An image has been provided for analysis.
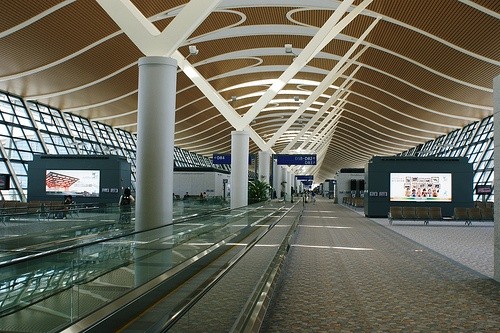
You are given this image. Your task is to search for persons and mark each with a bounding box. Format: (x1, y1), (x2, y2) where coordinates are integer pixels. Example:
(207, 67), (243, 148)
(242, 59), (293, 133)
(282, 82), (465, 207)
(173, 193), (177, 199)
(118, 188), (135, 224)
(54, 195), (74, 219)
(291, 187), (295, 203)
(304, 190), (316, 206)
(183, 192), (190, 200)
(405, 187), (438, 197)
(200, 192), (209, 203)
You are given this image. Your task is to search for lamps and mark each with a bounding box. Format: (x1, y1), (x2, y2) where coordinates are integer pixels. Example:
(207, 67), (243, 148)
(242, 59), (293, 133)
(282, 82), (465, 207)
(293, 96), (299, 101)
(253, 121), (256, 123)
(232, 95), (236, 101)
(186, 45), (199, 60)
(284, 43), (299, 60)
(299, 120), (302, 123)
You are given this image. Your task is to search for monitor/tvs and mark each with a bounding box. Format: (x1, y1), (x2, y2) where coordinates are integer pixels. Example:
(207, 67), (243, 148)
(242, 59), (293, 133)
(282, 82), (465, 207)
(0, 174), (10, 190)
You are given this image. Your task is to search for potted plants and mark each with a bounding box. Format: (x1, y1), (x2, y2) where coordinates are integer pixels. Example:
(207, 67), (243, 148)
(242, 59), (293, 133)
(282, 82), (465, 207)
(249, 180), (269, 204)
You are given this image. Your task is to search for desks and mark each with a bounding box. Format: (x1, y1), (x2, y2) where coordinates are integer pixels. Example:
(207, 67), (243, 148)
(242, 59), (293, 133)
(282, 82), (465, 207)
(0, 206), (42, 226)
(44, 204), (82, 220)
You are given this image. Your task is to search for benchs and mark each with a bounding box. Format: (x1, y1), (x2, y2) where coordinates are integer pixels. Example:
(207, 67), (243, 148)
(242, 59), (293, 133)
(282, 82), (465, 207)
(174, 195), (181, 200)
(1, 206), (99, 225)
(454, 202), (494, 226)
(343, 197), (365, 210)
(387, 207), (443, 226)
(185, 195), (200, 201)
